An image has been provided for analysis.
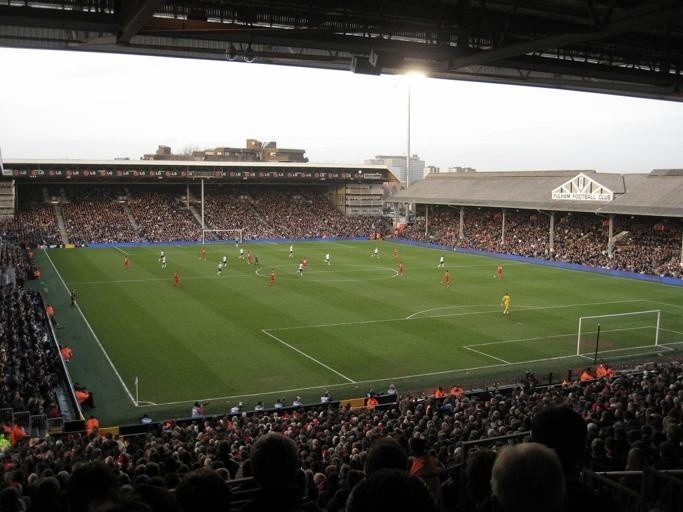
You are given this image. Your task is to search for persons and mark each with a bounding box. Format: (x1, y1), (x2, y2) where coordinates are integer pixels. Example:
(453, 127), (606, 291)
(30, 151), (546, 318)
(123, 256), (128, 268)
(296, 259), (306, 276)
(500, 292), (511, 316)
(1, 377), (682, 512)
(271, 271), (275, 283)
(393, 249), (397, 256)
(289, 245), (293, 259)
(216, 255), (227, 276)
(17, 172), (394, 245)
(398, 207), (683, 286)
(158, 250), (179, 283)
(235, 237), (260, 266)
(325, 252), (331, 266)
(1, 216), (42, 292)
(373, 248), (378, 257)
(200, 249), (206, 259)
(0, 285), (61, 377)
